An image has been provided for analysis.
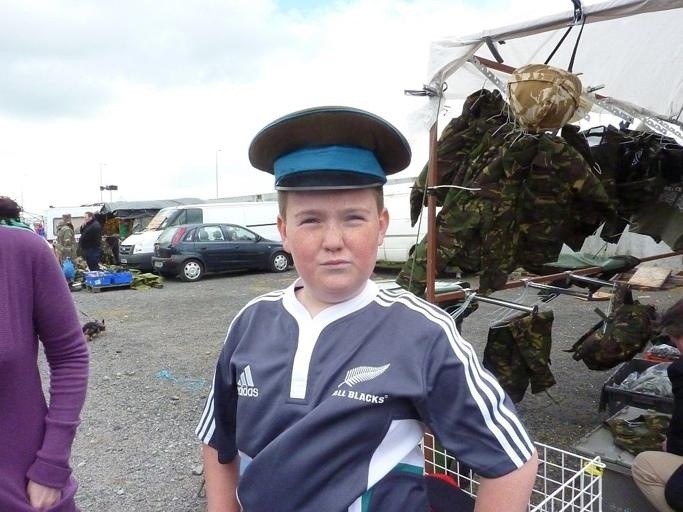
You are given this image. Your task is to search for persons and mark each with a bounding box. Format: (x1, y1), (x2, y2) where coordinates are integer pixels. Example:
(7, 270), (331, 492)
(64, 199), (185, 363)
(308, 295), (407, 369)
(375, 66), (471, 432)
(195, 104), (538, 512)
(80, 212), (102, 271)
(631, 300), (683, 512)
(36, 224), (45, 237)
(57, 214), (77, 284)
(0, 196), (92, 511)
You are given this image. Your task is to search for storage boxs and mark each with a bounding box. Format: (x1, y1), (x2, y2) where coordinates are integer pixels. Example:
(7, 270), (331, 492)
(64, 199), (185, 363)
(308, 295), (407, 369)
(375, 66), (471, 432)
(600, 358), (673, 418)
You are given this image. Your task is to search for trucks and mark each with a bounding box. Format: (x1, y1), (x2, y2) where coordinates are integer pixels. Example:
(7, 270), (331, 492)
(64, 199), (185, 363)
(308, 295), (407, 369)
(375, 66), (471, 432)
(374, 182), (444, 269)
(45, 205), (104, 248)
(116, 201), (280, 272)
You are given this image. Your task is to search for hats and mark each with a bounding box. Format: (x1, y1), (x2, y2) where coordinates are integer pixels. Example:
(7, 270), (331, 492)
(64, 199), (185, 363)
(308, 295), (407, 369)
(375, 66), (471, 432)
(249, 106), (410, 191)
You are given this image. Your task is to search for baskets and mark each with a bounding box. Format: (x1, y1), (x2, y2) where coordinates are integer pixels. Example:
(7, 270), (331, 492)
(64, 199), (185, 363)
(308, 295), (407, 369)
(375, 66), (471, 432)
(424, 421), (603, 512)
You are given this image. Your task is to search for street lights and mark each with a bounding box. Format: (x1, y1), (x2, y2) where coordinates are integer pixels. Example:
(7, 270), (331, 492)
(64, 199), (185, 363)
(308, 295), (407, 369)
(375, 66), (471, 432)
(99, 184), (118, 203)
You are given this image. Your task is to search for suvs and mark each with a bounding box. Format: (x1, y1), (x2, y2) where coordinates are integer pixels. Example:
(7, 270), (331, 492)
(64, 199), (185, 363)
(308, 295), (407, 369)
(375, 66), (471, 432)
(151, 223), (293, 282)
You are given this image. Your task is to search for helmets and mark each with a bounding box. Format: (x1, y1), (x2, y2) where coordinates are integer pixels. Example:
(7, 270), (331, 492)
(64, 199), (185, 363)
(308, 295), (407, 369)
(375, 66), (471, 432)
(507, 65), (582, 131)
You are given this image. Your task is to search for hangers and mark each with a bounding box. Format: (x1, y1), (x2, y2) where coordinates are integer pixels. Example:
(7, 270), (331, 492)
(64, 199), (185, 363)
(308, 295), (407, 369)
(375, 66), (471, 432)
(592, 209), (639, 239)
(444, 271), (556, 334)
(445, 75), (683, 175)
(601, 279), (618, 335)
